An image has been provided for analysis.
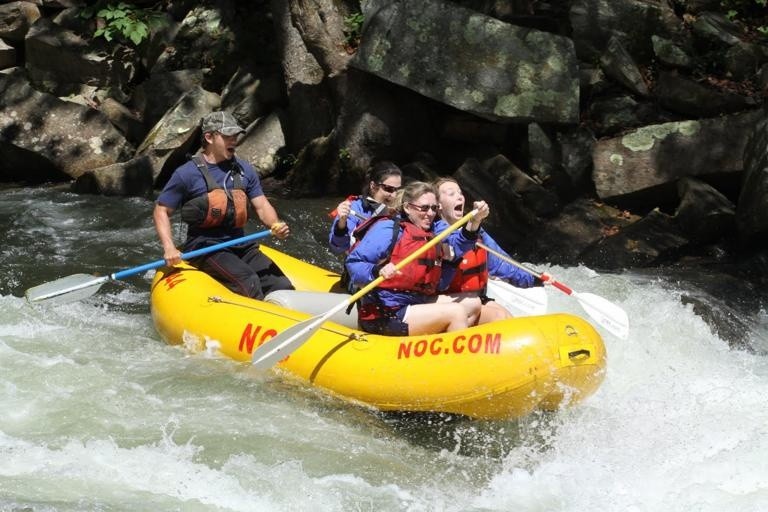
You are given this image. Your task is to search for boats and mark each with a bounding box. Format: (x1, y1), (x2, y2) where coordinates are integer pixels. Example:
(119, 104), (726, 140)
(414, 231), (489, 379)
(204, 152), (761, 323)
(144, 243), (609, 426)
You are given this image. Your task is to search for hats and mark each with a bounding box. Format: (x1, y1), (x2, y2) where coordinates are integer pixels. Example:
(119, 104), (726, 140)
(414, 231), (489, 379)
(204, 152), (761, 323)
(202, 111), (246, 136)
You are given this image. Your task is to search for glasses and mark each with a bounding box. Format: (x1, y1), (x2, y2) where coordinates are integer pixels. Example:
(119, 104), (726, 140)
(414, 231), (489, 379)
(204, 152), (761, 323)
(377, 182), (404, 193)
(408, 203), (438, 212)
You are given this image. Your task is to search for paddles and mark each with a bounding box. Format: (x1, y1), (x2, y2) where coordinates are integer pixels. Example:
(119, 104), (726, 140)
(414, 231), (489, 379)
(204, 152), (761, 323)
(474, 242), (631, 341)
(25, 221), (283, 313)
(251, 207), (479, 374)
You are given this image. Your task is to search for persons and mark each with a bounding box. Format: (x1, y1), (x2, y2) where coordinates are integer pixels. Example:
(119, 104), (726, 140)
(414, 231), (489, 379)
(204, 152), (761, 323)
(327, 159), (404, 293)
(429, 174), (554, 323)
(341, 181), (490, 335)
(154, 109), (294, 306)
